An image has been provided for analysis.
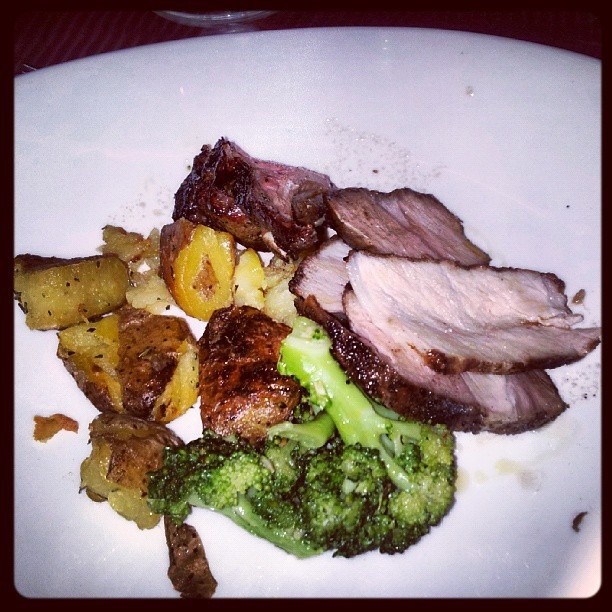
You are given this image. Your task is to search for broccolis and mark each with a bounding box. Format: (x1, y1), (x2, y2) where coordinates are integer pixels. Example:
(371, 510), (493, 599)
(146, 317), (457, 557)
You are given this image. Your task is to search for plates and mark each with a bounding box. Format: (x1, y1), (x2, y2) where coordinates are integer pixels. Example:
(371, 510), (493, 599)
(13, 25), (604, 600)
